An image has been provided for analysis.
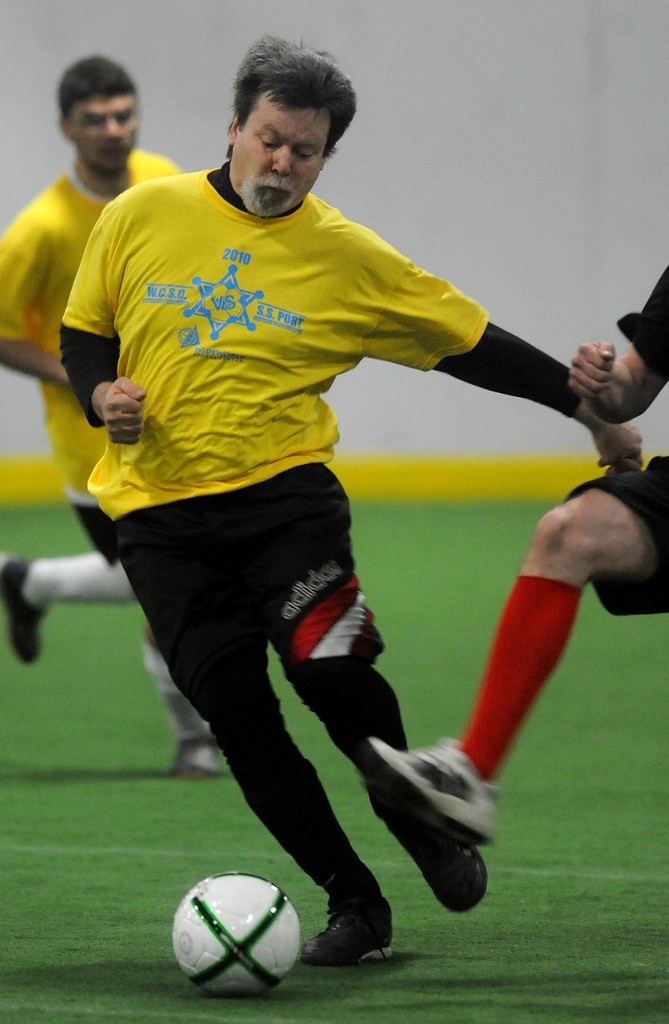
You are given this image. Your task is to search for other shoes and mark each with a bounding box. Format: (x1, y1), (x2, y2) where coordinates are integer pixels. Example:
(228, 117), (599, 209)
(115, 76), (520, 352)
(401, 821), (488, 911)
(298, 906), (395, 966)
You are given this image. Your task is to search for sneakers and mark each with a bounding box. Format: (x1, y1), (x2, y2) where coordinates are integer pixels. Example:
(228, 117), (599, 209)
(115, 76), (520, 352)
(361, 736), (501, 845)
(171, 739), (223, 777)
(0, 553), (40, 662)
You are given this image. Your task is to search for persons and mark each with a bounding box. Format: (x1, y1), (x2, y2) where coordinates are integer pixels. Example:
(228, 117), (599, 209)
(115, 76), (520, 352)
(361, 265), (668, 850)
(1, 52), (226, 779)
(57, 30), (647, 970)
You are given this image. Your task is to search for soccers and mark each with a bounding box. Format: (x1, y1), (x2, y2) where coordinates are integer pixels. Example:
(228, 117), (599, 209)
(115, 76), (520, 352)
(172, 870), (301, 997)
